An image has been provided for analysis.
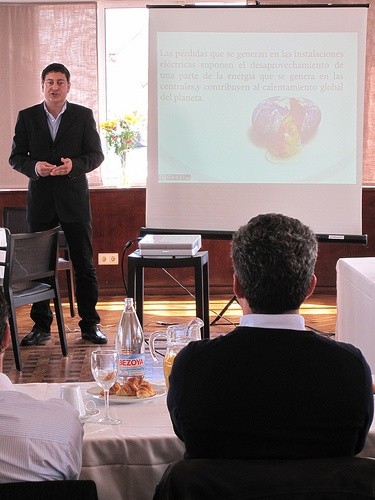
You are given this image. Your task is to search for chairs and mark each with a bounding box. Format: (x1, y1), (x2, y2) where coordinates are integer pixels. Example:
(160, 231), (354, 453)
(0, 207), (75, 371)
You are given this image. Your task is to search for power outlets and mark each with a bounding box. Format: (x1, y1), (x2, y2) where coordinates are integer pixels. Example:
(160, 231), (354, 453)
(98, 252), (119, 265)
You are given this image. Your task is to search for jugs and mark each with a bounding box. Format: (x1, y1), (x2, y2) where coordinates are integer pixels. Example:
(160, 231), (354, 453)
(149, 318), (204, 387)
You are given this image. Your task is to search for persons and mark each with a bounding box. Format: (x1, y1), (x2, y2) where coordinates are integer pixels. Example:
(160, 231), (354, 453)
(8, 63), (108, 346)
(166, 213), (374, 459)
(0, 285), (84, 483)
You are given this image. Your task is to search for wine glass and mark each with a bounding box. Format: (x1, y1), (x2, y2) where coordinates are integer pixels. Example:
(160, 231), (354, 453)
(91, 350), (123, 425)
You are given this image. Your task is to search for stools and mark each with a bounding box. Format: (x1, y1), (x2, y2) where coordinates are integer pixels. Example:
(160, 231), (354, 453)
(127, 249), (211, 342)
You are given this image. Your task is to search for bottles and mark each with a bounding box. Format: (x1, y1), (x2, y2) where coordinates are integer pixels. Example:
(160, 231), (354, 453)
(114, 297), (145, 385)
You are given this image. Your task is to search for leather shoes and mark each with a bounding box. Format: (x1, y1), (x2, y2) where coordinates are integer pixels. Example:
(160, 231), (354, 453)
(21, 328), (51, 346)
(81, 328), (108, 344)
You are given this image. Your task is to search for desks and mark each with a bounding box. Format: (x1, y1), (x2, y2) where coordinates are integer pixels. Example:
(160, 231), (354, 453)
(12, 380), (187, 500)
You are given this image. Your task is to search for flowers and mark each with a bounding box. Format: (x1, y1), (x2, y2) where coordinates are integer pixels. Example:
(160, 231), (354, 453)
(98, 114), (140, 167)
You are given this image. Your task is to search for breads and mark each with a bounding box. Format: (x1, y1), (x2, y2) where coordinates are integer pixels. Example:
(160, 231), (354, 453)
(100, 372), (155, 398)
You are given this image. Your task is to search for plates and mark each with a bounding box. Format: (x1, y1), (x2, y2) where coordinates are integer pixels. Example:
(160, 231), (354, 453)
(88, 384), (166, 404)
(80, 409), (100, 420)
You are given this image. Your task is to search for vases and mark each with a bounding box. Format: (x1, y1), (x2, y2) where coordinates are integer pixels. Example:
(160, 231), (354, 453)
(118, 148), (128, 187)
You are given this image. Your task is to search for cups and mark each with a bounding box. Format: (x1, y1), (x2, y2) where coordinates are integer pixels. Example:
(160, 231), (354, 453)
(61, 385), (87, 416)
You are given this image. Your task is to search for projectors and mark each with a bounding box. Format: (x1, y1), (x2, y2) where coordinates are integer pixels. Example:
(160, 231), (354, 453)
(139, 235), (201, 256)
